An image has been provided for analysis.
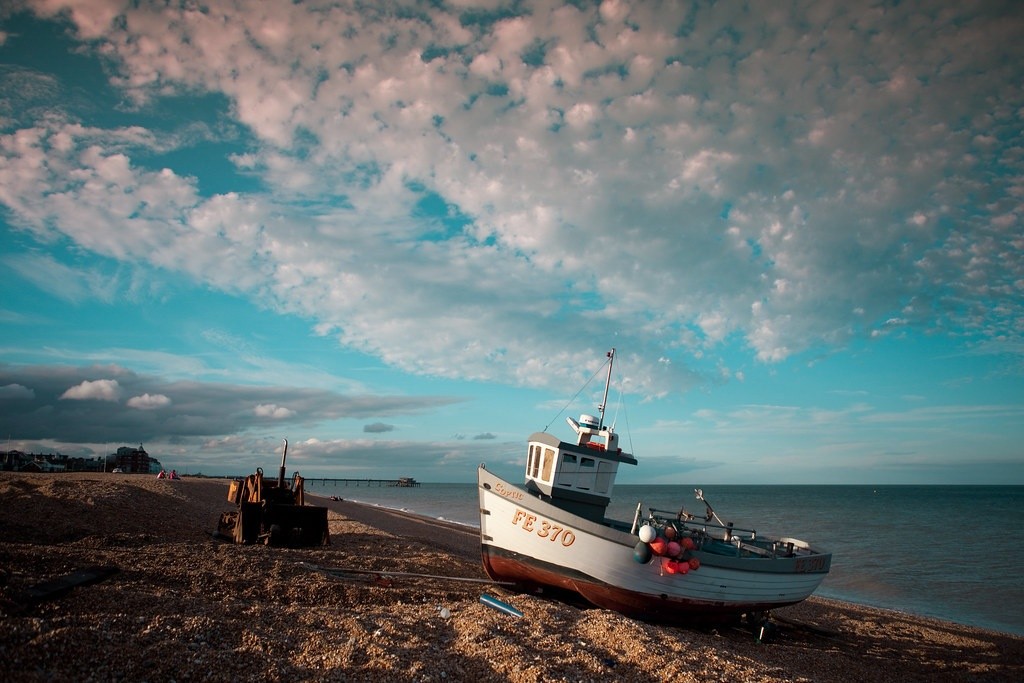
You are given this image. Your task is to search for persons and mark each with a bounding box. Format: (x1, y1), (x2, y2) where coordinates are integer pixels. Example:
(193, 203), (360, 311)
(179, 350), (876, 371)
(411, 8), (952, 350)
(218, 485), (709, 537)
(156, 469), (167, 479)
(169, 469), (179, 479)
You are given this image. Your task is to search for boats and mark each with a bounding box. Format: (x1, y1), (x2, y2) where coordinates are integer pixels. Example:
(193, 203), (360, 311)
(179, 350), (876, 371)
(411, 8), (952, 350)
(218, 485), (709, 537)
(478, 349), (833, 628)
(400, 477), (416, 483)
(113, 468), (123, 474)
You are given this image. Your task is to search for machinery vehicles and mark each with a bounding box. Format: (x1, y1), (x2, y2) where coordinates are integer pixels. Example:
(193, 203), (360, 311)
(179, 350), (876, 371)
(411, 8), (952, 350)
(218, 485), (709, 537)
(212, 438), (329, 549)
